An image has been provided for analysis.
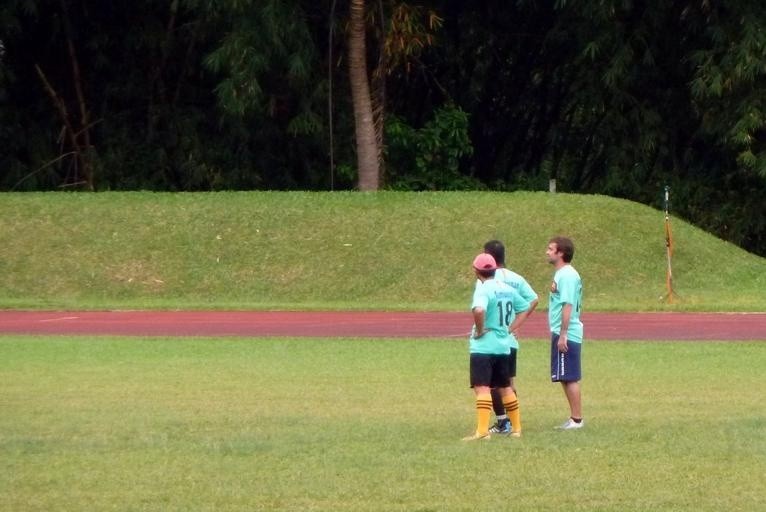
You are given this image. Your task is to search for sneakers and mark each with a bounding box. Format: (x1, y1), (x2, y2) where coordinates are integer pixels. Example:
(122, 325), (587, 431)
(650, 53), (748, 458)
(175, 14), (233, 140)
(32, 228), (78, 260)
(506, 430), (522, 439)
(487, 416), (513, 435)
(552, 416), (584, 432)
(458, 430), (492, 442)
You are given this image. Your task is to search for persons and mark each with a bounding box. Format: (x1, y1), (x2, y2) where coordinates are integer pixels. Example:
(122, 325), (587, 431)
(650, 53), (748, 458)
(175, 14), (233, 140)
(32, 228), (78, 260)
(546, 237), (586, 430)
(460, 253), (530, 443)
(475, 239), (539, 436)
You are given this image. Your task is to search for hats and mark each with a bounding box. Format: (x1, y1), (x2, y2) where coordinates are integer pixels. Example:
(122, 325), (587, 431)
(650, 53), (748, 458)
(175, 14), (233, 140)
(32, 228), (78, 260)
(471, 252), (499, 272)
